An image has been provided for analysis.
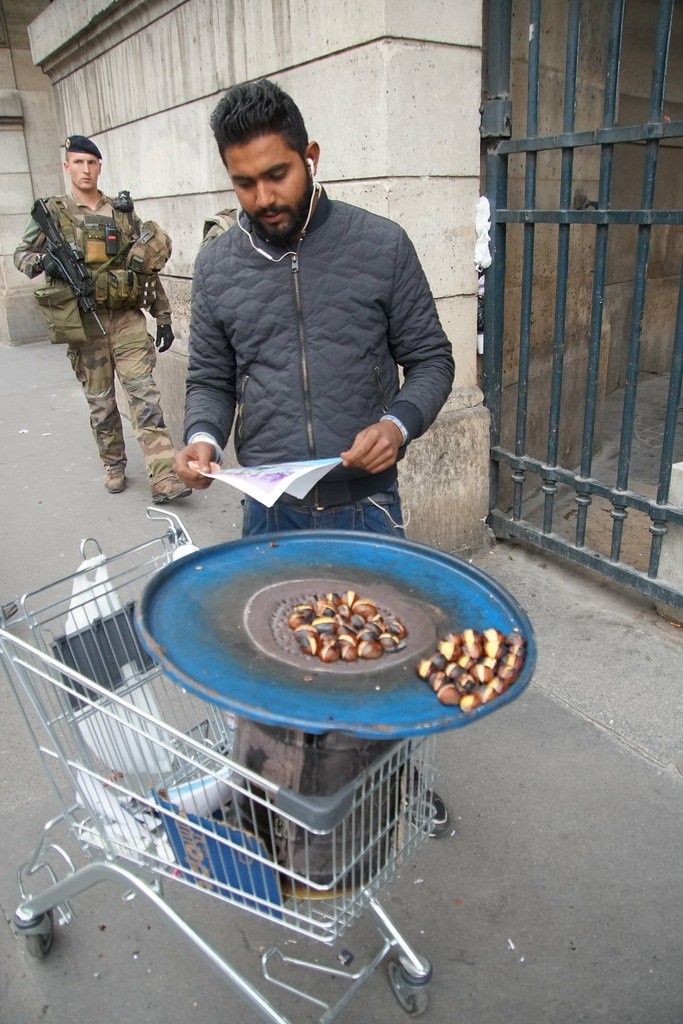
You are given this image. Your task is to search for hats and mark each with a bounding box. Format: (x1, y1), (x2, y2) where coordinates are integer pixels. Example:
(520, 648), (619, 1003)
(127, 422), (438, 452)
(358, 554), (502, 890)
(65, 135), (102, 160)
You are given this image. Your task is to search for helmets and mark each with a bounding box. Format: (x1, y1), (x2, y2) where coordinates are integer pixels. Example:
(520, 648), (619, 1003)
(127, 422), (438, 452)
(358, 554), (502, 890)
(126, 221), (173, 275)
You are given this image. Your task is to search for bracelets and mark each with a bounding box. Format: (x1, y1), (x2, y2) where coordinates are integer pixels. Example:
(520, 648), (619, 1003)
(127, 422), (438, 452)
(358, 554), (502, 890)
(189, 436), (224, 465)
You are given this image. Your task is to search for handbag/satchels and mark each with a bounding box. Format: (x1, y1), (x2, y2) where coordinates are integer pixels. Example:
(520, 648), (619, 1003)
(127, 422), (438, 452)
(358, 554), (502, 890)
(34, 284), (87, 344)
(65, 554), (176, 775)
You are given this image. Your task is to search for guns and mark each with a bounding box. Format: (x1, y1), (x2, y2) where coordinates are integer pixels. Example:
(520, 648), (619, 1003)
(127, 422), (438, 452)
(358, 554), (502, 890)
(30, 197), (109, 336)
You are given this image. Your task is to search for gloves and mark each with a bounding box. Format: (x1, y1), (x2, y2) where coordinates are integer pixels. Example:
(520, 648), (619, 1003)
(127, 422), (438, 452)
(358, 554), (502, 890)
(41, 253), (68, 282)
(156, 324), (174, 353)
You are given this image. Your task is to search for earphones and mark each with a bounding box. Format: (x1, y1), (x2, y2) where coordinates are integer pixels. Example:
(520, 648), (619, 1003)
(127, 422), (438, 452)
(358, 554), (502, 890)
(307, 158), (314, 174)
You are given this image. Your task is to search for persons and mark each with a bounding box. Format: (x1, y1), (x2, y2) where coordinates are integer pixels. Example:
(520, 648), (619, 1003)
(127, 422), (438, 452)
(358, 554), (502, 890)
(173, 80), (455, 838)
(13, 135), (192, 504)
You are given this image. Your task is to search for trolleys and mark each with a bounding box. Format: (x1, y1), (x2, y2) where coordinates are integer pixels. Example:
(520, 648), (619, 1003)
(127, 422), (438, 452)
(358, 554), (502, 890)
(0, 507), (438, 1024)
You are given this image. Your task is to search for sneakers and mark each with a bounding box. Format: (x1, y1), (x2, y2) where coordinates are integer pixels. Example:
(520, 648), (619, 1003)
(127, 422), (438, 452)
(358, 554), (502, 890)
(103, 467), (126, 493)
(150, 477), (193, 503)
(398, 764), (451, 837)
